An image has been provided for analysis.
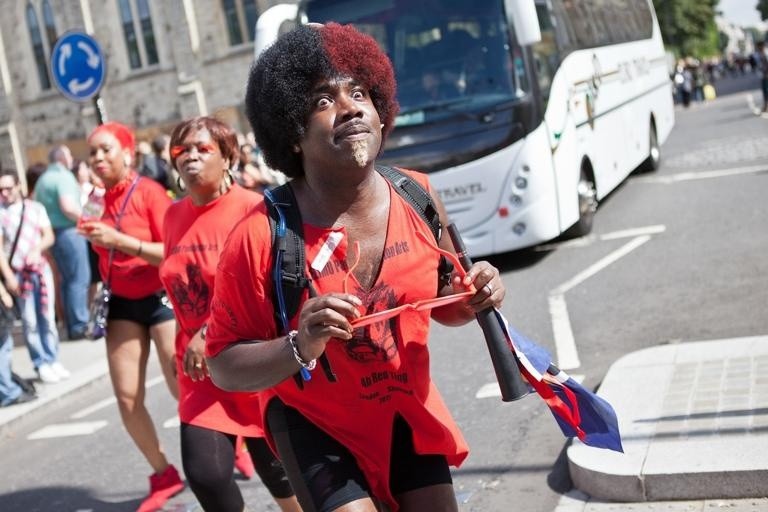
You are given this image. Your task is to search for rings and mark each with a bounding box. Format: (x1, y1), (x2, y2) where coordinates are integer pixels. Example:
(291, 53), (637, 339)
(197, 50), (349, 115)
(483, 282), (494, 294)
(195, 362), (202, 369)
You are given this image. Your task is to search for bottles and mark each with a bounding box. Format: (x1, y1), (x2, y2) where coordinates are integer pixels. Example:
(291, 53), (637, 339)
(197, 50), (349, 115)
(75, 185), (107, 241)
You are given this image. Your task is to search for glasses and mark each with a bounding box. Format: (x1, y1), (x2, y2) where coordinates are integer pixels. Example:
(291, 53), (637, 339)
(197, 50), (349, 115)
(342, 231), (478, 330)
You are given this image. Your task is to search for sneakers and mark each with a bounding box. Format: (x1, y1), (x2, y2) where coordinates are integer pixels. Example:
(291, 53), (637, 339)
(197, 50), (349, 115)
(137, 466), (183, 512)
(236, 446), (253, 479)
(10, 363), (68, 403)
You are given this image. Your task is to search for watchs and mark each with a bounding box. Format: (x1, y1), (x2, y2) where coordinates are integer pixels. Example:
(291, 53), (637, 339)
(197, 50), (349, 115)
(198, 323), (211, 337)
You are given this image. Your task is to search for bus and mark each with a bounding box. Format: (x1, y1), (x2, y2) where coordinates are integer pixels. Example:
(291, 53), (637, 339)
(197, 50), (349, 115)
(255, 0), (676, 259)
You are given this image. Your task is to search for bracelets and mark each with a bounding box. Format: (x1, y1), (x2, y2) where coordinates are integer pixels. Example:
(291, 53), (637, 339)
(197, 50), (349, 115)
(135, 238), (144, 257)
(285, 327), (318, 372)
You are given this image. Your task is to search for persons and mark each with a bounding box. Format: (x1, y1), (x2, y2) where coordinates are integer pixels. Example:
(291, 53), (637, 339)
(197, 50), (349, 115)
(669, 39), (768, 117)
(1, 169), (73, 405)
(77, 120), (255, 511)
(154, 115), (302, 511)
(27, 134), (286, 340)
(198, 19), (510, 512)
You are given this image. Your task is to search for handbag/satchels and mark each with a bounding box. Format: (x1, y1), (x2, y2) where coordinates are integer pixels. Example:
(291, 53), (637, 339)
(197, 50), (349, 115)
(86, 288), (110, 339)
